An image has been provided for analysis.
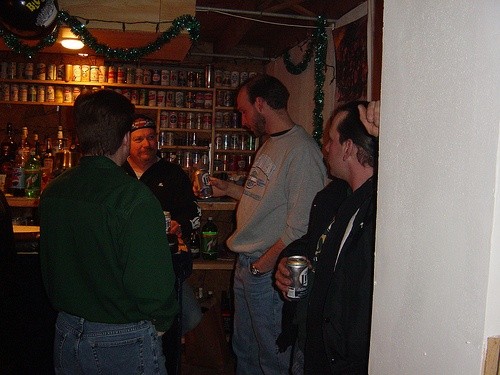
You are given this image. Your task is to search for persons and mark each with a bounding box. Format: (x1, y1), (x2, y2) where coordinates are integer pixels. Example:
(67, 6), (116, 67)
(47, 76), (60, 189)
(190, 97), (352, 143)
(39, 90), (179, 375)
(120, 114), (198, 375)
(274, 99), (381, 375)
(192, 74), (325, 375)
(0, 189), (18, 375)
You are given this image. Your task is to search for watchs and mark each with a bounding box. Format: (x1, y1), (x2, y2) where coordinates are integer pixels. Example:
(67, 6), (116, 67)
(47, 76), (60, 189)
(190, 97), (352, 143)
(250, 263), (267, 276)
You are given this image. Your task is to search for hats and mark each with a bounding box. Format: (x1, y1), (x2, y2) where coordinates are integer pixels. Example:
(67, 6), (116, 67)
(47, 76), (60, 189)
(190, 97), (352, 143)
(129, 113), (158, 133)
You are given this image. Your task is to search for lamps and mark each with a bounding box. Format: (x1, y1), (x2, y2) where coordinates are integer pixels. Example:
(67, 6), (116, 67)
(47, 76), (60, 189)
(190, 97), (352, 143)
(60, 29), (84, 50)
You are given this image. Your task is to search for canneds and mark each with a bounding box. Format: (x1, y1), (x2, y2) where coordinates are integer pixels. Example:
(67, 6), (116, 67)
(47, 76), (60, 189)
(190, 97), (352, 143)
(160, 131), (261, 171)
(164, 212), (171, 234)
(0, 60), (257, 109)
(160, 111), (242, 130)
(195, 168), (213, 198)
(285, 255), (309, 299)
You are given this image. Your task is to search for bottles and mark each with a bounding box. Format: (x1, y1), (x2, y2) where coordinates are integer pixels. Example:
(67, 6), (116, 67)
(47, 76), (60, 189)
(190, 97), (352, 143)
(1, 122), (80, 199)
(189, 229), (200, 257)
(201, 217), (219, 259)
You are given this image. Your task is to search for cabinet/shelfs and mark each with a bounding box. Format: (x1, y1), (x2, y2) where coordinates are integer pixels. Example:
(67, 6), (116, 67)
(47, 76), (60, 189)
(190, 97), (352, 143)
(0, 78), (258, 178)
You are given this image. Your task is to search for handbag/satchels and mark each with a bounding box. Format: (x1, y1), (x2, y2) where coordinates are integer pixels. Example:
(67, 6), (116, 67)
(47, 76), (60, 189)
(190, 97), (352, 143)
(183, 299), (232, 369)
